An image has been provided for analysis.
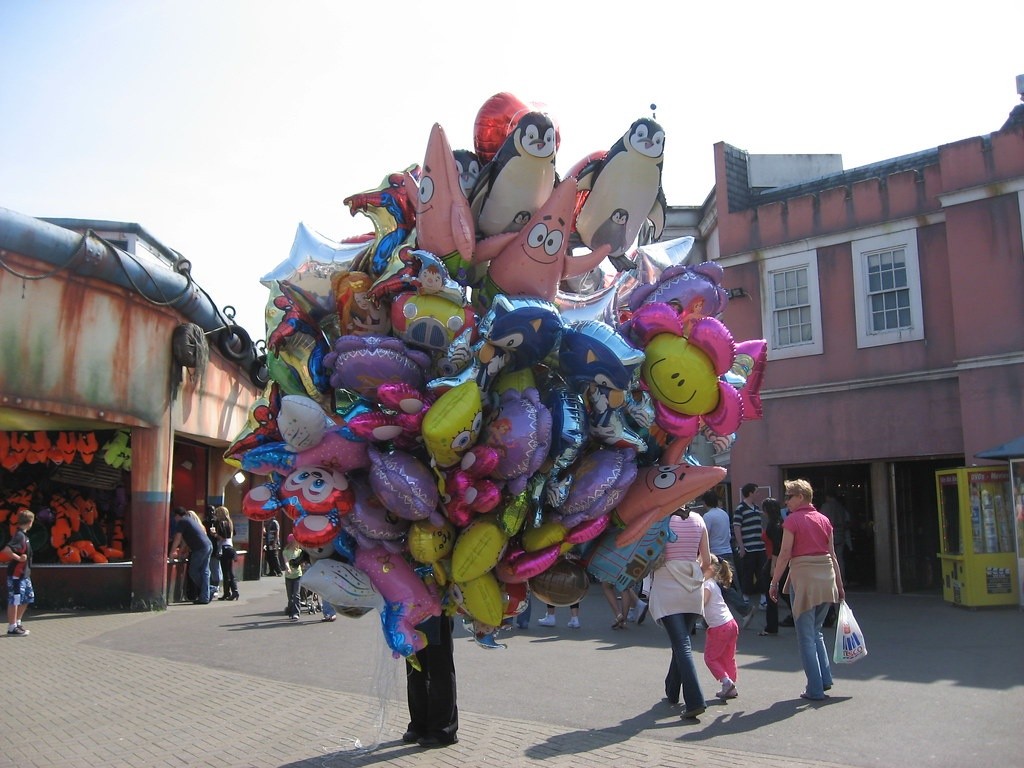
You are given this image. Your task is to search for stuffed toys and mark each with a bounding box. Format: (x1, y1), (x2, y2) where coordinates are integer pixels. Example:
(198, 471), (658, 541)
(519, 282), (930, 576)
(0, 429), (131, 563)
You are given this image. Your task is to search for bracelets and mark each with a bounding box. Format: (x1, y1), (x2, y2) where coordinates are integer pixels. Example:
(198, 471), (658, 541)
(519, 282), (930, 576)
(770, 583), (778, 588)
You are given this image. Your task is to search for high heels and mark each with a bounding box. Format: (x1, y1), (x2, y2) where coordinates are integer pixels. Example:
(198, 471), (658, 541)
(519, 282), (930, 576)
(226, 591), (239, 600)
(218, 592), (231, 600)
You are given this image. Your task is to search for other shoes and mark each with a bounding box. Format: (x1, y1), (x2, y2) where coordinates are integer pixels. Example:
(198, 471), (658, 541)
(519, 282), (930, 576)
(611, 614), (626, 628)
(738, 594), (795, 636)
(7, 625), (30, 637)
(194, 600), (208, 604)
(681, 707), (706, 717)
(715, 682), (738, 699)
(322, 613), (336, 621)
(696, 622), (707, 629)
(418, 735), (447, 747)
(402, 730), (424, 742)
(800, 690), (809, 698)
(636, 602), (648, 624)
(268, 572), (277, 576)
(823, 683), (831, 691)
(627, 615), (635, 622)
(277, 572), (283, 577)
(289, 613), (299, 620)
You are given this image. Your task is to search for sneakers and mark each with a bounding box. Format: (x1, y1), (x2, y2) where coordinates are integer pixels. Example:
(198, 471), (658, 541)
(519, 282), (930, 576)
(538, 616), (556, 626)
(567, 620), (580, 627)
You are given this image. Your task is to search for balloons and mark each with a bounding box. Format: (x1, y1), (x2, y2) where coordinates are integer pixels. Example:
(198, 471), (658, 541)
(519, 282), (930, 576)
(218, 92), (771, 671)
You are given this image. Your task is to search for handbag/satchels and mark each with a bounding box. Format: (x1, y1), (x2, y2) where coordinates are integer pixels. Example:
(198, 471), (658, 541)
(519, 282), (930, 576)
(833, 600), (868, 663)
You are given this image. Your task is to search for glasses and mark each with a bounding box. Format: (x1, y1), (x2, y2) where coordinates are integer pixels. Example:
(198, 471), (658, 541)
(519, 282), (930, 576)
(785, 494), (798, 501)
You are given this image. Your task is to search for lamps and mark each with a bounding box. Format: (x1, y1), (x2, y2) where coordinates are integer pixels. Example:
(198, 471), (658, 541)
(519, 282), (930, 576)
(731, 288), (745, 297)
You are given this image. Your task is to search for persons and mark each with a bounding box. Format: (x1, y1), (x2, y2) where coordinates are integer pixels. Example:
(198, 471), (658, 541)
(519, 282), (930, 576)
(282, 534), (337, 622)
(644, 481), (797, 720)
(263, 516), (283, 577)
(403, 608), (460, 747)
(3, 509), (34, 637)
(503, 579), (649, 629)
(769, 478), (844, 701)
(169, 505), (239, 604)
